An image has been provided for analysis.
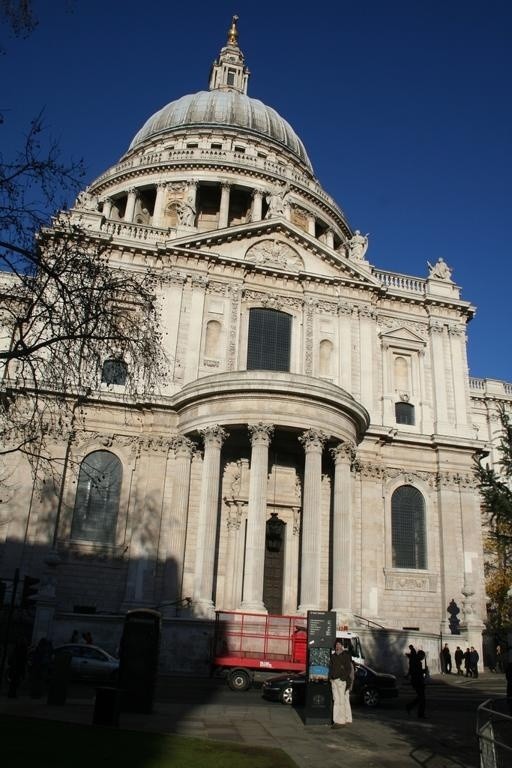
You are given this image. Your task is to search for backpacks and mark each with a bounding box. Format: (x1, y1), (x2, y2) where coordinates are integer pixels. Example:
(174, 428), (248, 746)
(348, 661), (354, 691)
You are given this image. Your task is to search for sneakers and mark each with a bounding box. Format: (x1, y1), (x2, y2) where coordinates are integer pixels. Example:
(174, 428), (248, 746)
(331, 722), (352, 729)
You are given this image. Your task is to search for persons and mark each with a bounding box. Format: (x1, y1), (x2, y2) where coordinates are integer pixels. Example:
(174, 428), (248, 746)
(349, 229), (367, 261)
(427, 256), (451, 280)
(405, 644), (416, 676)
(469, 646), (479, 679)
(454, 647), (464, 675)
(341, 648), (356, 723)
(328, 643), (351, 730)
(442, 643), (452, 674)
(410, 649), (429, 718)
(177, 196), (198, 227)
(462, 648), (472, 677)
(265, 180), (287, 218)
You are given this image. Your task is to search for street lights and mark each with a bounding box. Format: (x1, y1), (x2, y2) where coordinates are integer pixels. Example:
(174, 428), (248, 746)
(50, 389), (90, 549)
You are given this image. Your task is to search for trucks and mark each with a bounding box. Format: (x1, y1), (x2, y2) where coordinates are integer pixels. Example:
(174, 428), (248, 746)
(214, 610), (368, 692)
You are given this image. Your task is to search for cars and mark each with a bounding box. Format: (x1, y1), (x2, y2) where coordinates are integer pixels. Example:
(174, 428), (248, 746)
(261, 663), (400, 708)
(44, 643), (119, 673)
